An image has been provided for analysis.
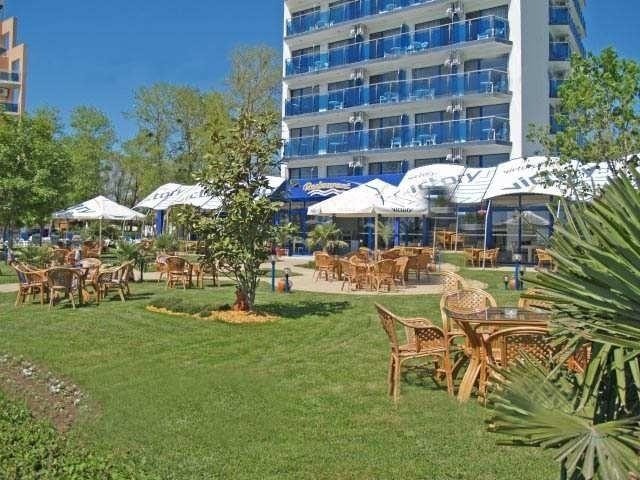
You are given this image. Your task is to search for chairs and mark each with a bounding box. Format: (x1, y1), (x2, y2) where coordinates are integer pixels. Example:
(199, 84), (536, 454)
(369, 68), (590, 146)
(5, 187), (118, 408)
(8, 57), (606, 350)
(463, 245), (500, 267)
(535, 248), (554, 270)
(440, 287), (592, 404)
(431, 228), (464, 252)
(315, 243), (435, 296)
(372, 302), (457, 404)
(11, 240), (219, 311)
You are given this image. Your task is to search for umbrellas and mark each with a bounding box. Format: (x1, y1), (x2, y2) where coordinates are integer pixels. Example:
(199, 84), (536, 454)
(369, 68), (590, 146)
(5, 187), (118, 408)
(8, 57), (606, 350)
(49, 194), (147, 256)
(304, 175), (436, 256)
